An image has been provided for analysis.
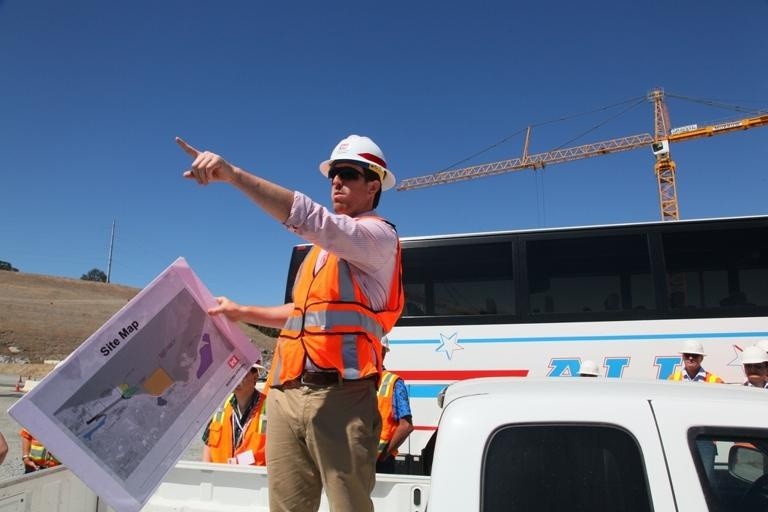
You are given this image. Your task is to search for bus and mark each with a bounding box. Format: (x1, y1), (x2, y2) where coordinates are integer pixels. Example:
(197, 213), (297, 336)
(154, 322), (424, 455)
(282, 213), (768, 467)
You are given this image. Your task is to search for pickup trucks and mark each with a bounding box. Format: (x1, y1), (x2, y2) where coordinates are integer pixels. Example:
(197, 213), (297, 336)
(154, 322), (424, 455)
(0, 371), (768, 511)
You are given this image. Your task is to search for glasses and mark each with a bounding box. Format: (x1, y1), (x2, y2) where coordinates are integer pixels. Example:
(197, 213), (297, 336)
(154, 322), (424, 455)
(328, 165), (365, 182)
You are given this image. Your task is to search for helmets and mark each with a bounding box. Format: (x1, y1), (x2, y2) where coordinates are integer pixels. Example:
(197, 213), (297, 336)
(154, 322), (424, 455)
(577, 360), (601, 376)
(678, 341), (712, 356)
(742, 348), (768, 364)
(320, 135), (396, 192)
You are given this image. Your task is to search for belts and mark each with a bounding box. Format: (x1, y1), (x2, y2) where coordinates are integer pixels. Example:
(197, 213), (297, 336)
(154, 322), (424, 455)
(281, 371), (341, 389)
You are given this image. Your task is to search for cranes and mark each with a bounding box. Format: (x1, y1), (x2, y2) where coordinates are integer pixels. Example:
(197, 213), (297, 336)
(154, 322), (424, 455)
(394, 84), (768, 222)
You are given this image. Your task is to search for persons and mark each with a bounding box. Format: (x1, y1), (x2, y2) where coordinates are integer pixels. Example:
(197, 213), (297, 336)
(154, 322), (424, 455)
(733, 347), (768, 449)
(19, 428), (59, 475)
(376, 333), (414, 474)
(201, 354), (268, 466)
(667, 340), (724, 483)
(172, 133), (405, 512)
(0, 432), (8, 465)
